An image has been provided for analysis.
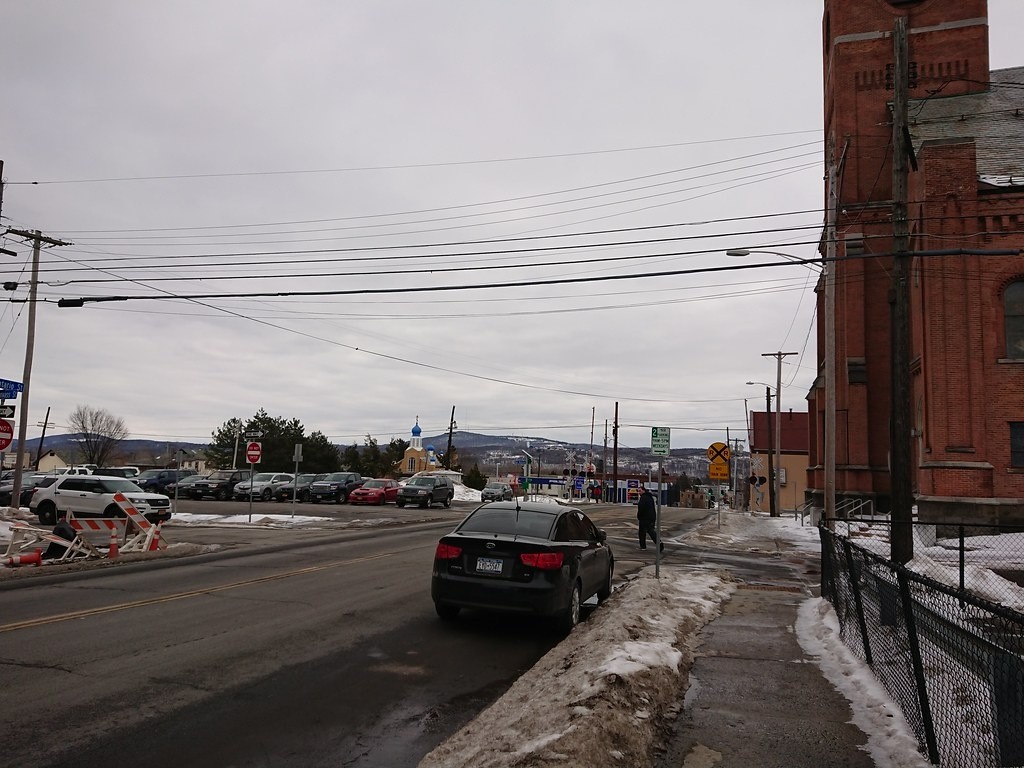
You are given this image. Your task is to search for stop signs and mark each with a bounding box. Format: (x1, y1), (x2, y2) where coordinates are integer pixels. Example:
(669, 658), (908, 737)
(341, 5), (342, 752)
(247, 443), (260, 462)
(0, 419), (13, 450)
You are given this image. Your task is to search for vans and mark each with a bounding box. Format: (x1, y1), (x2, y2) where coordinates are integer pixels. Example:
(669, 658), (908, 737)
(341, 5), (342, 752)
(128, 469), (199, 494)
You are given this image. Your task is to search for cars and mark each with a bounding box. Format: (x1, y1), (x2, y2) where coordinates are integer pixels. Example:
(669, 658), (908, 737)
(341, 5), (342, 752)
(430, 502), (614, 628)
(481, 482), (514, 502)
(163, 475), (207, 498)
(233, 472), (316, 502)
(349, 478), (402, 505)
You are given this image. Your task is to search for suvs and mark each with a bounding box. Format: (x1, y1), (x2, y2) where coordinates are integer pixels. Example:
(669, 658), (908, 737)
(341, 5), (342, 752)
(309, 472), (364, 504)
(189, 468), (259, 502)
(395, 473), (455, 508)
(0, 464), (173, 525)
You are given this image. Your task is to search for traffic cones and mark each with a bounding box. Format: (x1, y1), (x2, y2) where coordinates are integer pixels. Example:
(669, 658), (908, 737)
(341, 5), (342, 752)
(0, 547), (42, 567)
(149, 520), (163, 551)
(107, 526), (122, 558)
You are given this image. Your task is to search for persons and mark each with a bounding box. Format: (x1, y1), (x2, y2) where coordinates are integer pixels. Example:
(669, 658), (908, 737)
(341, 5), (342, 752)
(636, 487), (664, 552)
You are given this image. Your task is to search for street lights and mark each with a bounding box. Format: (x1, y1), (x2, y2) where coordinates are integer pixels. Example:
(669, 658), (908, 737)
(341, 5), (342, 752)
(745, 381), (782, 517)
(726, 249), (838, 532)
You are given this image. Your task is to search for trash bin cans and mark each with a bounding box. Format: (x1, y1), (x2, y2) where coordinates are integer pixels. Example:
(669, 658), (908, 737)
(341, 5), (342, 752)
(633, 496), (639, 505)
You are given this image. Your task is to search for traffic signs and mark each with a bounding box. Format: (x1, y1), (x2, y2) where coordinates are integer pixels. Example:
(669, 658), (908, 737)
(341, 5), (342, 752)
(0, 404), (15, 418)
(651, 427), (671, 456)
(244, 429), (265, 439)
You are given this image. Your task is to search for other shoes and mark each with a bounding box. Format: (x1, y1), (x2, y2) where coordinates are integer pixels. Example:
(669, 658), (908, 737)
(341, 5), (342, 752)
(637, 548), (647, 550)
(659, 542), (665, 552)
